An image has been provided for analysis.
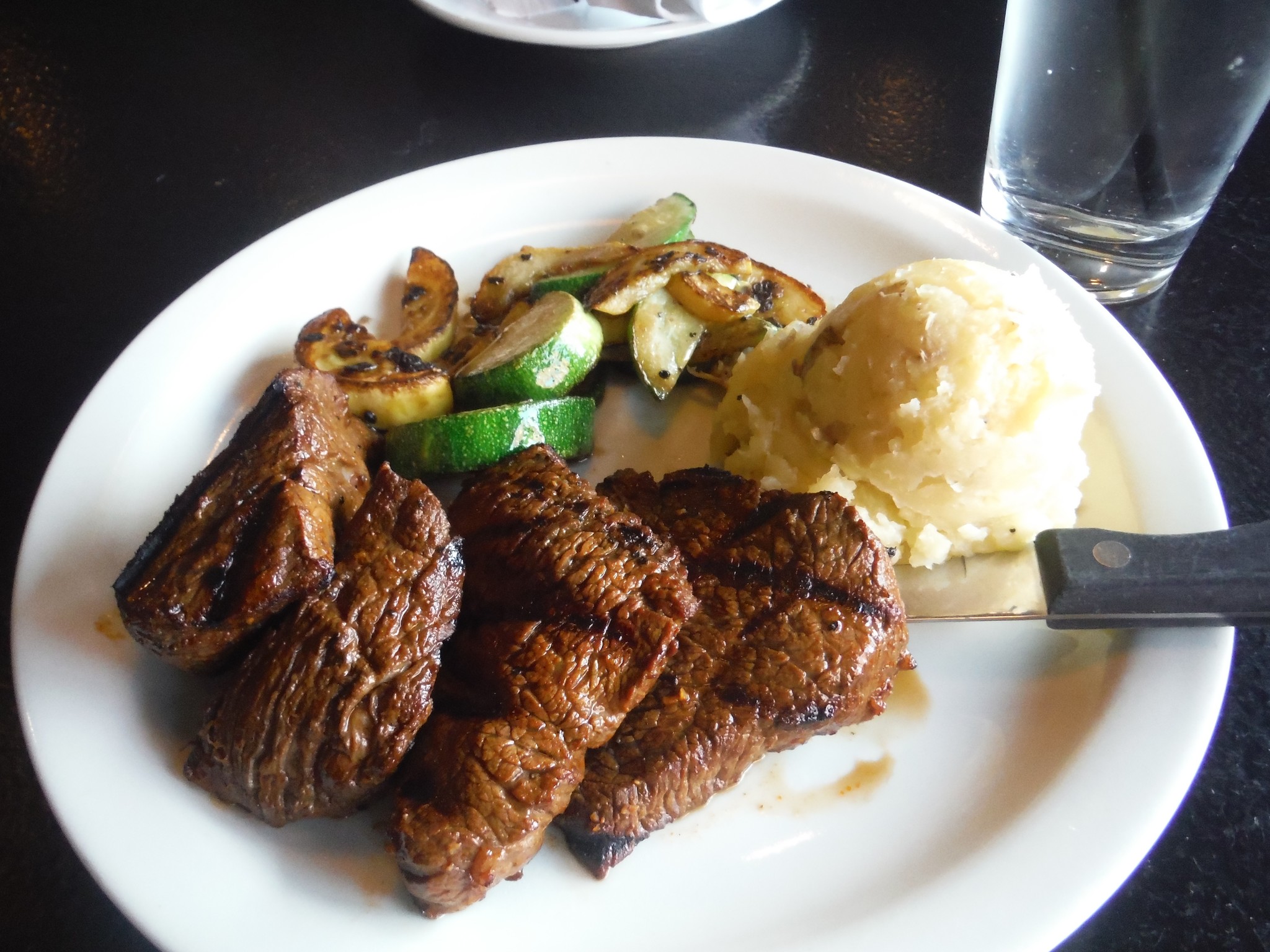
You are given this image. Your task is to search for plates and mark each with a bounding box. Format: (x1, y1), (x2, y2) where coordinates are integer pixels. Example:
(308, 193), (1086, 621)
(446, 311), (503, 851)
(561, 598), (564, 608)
(414, 1), (783, 49)
(10, 137), (1238, 952)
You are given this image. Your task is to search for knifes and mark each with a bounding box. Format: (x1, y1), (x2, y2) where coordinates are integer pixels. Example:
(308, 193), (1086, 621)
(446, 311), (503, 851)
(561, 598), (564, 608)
(893, 518), (1270, 629)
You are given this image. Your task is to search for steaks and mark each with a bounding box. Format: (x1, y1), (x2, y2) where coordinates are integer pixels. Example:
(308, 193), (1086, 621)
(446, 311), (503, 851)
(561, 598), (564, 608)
(108, 367), (908, 908)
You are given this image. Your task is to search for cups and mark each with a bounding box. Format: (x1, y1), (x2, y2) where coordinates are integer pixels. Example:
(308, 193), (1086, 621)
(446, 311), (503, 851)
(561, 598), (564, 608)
(980, 0), (1270, 305)
